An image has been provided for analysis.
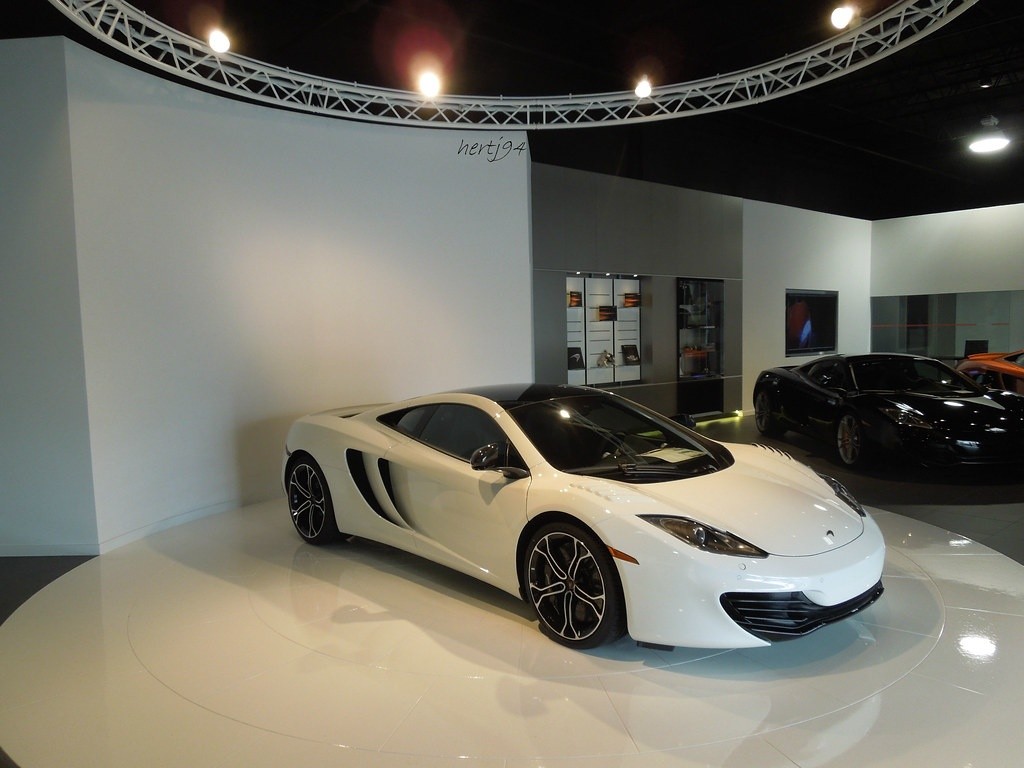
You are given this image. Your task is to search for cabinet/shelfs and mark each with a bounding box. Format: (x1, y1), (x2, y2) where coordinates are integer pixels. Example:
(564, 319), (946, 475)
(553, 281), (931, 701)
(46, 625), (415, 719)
(676, 277), (725, 417)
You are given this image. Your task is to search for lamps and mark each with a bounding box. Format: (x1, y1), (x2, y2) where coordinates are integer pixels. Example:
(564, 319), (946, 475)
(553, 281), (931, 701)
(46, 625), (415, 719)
(830, 5), (862, 29)
(634, 73), (652, 98)
(969, 115), (1010, 152)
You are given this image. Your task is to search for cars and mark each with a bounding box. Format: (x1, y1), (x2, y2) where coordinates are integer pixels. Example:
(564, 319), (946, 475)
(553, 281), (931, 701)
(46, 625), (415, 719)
(955, 349), (1024, 398)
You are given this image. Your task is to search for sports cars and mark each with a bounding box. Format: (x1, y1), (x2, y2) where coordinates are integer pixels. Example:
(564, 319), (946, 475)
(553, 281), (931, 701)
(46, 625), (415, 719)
(753, 352), (1024, 478)
(280, 383), (887, 650)
